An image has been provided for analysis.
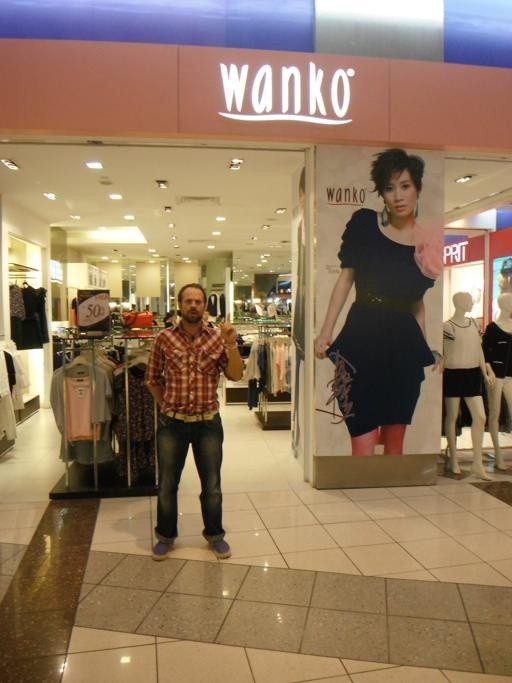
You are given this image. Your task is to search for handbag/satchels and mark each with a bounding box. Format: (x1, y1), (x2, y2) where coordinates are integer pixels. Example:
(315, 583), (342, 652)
(122, 310), (155, 336)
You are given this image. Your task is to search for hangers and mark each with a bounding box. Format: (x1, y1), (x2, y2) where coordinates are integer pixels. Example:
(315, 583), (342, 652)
(246, 324), (295, 347)
(8, 279), (42, 289)
(52, 341), (151, 379)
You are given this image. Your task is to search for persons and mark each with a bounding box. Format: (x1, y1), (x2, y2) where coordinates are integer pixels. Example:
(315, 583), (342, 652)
(312, 146), (436, 455)
(442, 290), (494, 481)
(142, 283), (244, 562)
(290, 162), (311, 463)
(481, 291), (511, 473)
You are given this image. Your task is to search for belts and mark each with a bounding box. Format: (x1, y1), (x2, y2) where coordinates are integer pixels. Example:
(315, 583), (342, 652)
(166, 410), (221, 422)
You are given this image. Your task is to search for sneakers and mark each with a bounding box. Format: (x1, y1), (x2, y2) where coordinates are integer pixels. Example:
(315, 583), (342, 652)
(153, 535), (171, 561)
(209, 534), (231, 558)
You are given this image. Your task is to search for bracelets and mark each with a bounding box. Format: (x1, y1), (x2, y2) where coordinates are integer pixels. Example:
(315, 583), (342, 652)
(225, 342), (238, 349)
